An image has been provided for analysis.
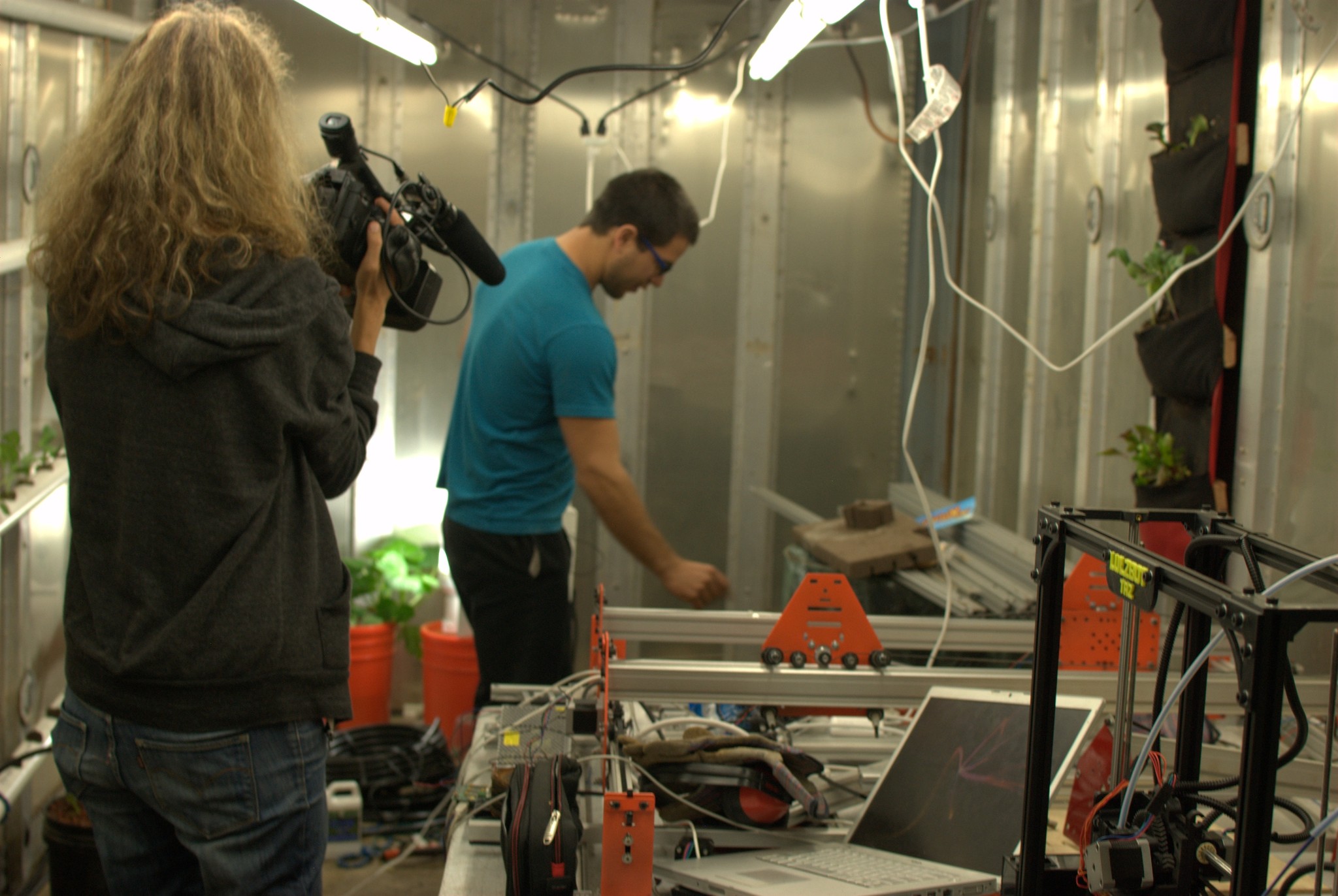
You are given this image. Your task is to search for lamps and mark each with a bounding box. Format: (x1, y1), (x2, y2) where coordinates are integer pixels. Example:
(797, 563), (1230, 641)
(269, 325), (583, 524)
(292, 0), (444, 69)
(744, 0), (866, 80)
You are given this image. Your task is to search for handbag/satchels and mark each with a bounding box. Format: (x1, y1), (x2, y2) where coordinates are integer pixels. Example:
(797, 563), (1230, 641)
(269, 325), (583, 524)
(500, 757), (586, 896)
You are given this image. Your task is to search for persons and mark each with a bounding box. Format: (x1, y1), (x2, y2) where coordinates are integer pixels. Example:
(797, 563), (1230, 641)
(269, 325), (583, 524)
(26, 3), (405, 896)
(436, 168), (731, 727)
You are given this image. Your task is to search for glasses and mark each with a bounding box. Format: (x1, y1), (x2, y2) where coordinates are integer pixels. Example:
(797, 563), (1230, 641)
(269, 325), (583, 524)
(643, 241), (676, 278)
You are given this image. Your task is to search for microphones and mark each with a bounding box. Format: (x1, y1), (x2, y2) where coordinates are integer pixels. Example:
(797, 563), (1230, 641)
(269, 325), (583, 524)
(438, 209), (509, 287)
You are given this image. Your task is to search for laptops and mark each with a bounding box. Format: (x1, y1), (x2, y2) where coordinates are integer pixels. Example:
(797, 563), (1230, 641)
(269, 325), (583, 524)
(653, 685), (1104, 896)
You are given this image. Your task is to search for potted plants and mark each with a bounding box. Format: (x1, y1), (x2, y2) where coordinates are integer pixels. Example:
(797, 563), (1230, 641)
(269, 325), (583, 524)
(332, 534), (433, 733)
(1144, 116), (1226, 236)
(1092, 424), (1208, 510)
(1111, 245), (1215, 401)
(421, 547), (479, 749)
